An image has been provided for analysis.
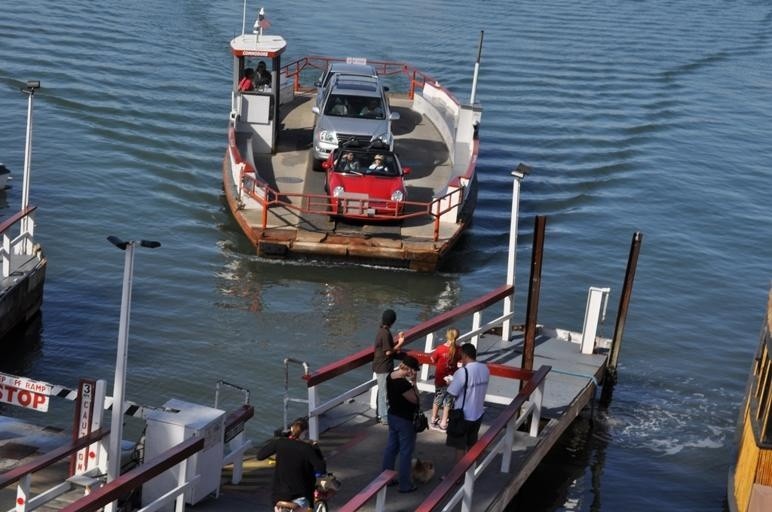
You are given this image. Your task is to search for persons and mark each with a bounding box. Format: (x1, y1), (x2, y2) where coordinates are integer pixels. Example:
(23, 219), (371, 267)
(360, 100), (383, 117)
(271, 416), (316, 511)
(430, 327), (462, 430)
(372, 309), (405, 425)
(366, 155), (388, 176)
(237, 68), (255, 92)
(440, 343), (489, 484)
(333, 152), (359, 171)
(254, 61), (272, 88)
(382, 355), (421, 492)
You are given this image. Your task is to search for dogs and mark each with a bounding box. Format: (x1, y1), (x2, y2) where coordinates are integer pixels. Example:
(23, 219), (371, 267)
(411, 456), (436, 481)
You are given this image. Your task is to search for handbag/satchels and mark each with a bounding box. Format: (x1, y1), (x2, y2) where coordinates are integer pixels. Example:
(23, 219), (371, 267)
(446, 409), (465, 436)
(417, 410), (428, 432)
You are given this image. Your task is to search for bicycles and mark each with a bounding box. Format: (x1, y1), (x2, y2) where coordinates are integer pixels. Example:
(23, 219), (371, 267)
(274, 474), (342, 512)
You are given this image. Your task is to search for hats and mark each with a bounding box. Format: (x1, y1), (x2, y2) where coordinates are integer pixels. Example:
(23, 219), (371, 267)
(382, 310), (396, 324)
(403, 355), (421, 371)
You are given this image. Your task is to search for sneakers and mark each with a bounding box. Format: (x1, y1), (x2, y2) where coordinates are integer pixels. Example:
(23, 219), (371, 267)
(431, 415), (440, 426)
(398, 486), (418, 494)
(387, 479), (399, 487)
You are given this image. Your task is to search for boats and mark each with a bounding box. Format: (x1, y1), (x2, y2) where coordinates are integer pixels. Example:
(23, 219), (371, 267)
(223, 0), (484, 274)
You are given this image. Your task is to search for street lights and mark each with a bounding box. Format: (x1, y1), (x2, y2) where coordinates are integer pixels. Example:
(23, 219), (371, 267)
(502, 162), (531, 341)
(18, 80), (41, 254)
(103, 236), (161, 512)
